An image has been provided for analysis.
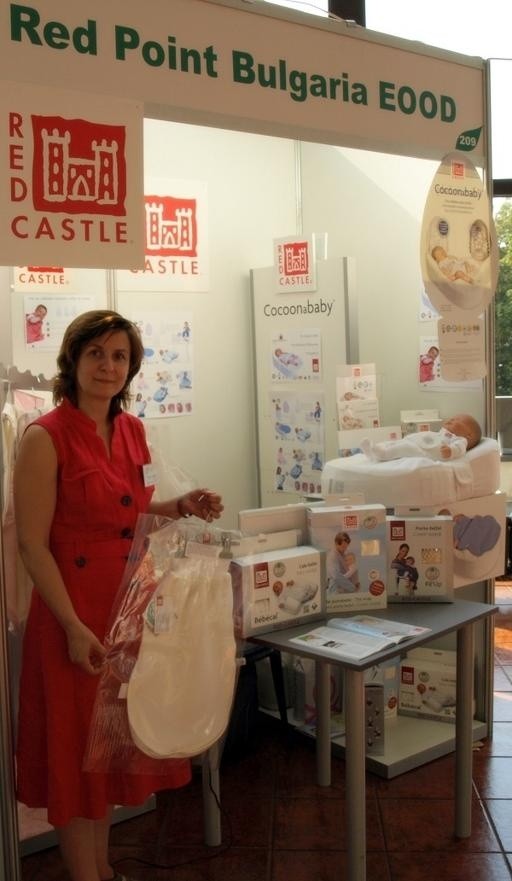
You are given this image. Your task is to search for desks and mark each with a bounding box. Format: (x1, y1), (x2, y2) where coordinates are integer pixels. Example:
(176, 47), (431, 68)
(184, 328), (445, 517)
(191, 597), (497, 881)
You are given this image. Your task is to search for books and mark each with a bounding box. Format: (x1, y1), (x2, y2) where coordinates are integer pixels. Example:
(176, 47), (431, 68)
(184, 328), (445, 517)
(288, 612), (433, 659)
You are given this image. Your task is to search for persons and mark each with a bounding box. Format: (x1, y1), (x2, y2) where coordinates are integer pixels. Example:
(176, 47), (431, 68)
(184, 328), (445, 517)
(13, 311), (224, 881)
(28, 306), (48, 343)
(389, 542), (418, 596)
(275, 349), (302, 367)
(419, 345), (439, 385)
(360, 407), (485, 460)
(342, 390), (367, 456)
(274, 399), (322, 494)
(134, 319), (193, 419)
(274, 580), (319, 609)
(325, 529), (358, 595)
(438, 509), (500, 555)
(417, 682), (456, 708)
(432, 242), (476, 292)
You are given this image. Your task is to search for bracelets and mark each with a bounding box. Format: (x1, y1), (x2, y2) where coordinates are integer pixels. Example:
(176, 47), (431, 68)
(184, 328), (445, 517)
(176, 495), (193, 520)
